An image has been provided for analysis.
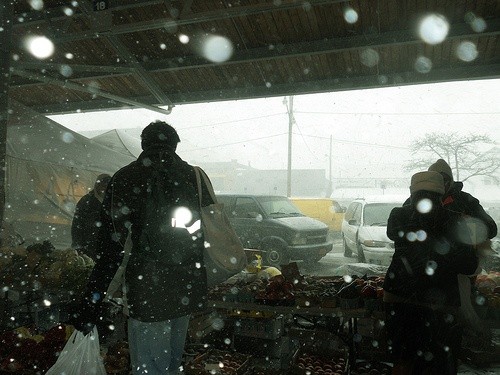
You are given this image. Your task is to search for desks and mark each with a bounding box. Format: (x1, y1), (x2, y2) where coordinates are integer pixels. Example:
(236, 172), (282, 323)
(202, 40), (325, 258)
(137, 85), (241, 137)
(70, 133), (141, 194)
(200, 272), (391, 372)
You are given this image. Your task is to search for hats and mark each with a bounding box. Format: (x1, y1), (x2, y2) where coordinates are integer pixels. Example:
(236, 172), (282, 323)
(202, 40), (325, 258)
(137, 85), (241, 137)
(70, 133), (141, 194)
(409, 171), (445, 195)
(428, 159), (452, 180)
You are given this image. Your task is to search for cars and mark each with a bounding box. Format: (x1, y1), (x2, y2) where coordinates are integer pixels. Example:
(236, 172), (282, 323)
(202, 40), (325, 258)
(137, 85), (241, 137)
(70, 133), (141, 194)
(342, 196), (410, 266)
(214, 192), (332, 270)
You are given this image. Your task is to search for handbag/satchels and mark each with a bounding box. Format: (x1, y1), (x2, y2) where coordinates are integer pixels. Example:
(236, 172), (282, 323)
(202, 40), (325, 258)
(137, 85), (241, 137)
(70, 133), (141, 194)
(192, 165), (246, 287)
(43, 326), (108, 374)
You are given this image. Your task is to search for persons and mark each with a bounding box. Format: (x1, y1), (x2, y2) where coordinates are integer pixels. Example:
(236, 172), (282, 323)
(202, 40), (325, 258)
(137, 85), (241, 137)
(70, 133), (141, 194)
(402, 158), (498, 366)
(70, 174), (113, 263)
(90, 120), (215, 375)
(384, 171), (479, 375)
